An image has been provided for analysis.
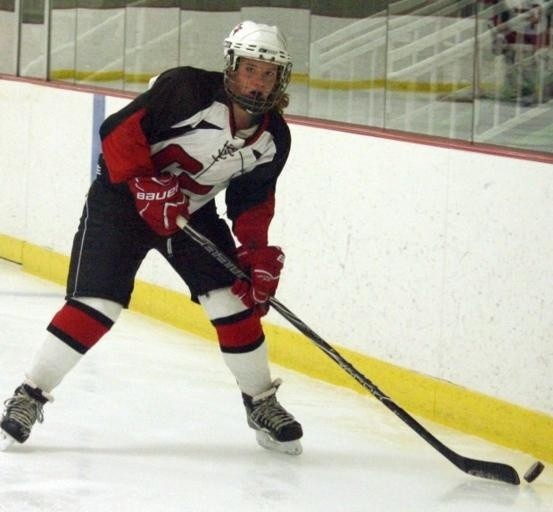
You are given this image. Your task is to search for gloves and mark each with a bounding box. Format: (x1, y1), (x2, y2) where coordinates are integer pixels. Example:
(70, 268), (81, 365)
(127, 172), (189, 237)
(231, 246), (284, 316)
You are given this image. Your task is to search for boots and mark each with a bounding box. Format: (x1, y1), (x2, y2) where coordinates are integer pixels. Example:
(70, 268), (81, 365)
(242, 378), (302, 442)
(0, 384), (47, 442)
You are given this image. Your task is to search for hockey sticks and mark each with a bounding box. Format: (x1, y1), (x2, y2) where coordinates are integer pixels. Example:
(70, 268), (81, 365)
(176, 214), (520, 485)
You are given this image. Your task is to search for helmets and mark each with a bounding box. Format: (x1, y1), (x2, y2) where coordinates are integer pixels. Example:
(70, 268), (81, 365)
(224, 20), (292, 76)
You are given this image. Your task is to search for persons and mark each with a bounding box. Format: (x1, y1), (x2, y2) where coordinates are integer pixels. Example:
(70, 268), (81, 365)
(0, 21), (302, 459)
(486, 0), (553, 106)
